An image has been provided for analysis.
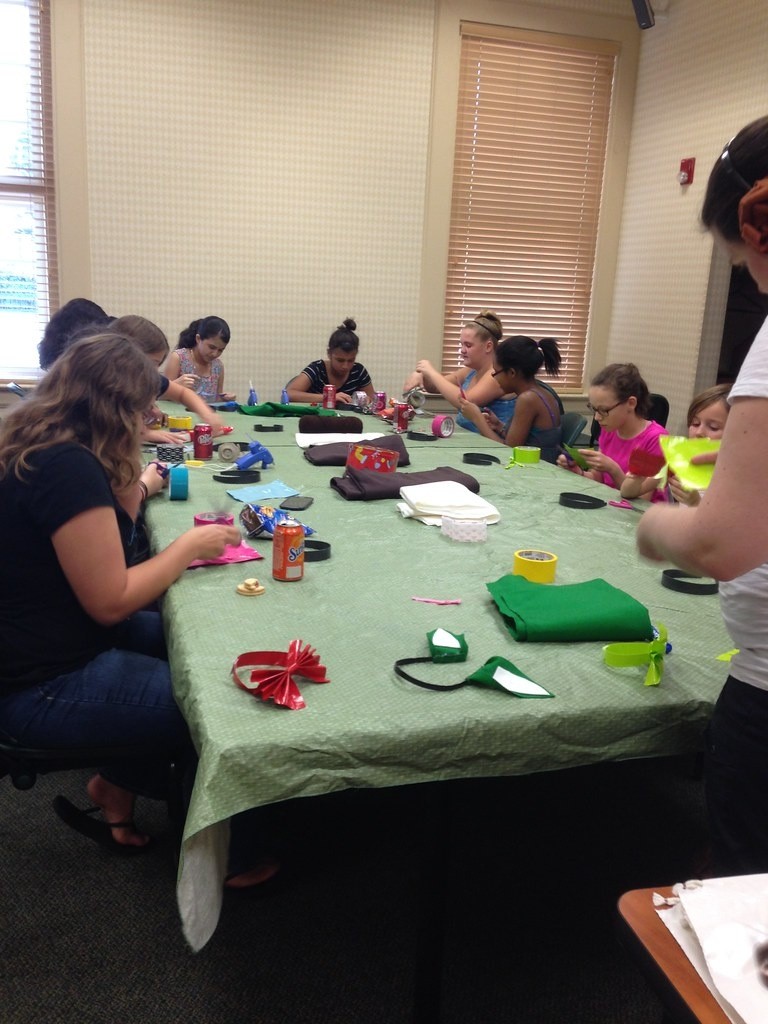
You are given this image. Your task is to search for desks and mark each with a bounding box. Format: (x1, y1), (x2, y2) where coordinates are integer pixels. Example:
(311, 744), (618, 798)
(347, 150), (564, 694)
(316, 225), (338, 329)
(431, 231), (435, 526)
(141, 401), (742, 1024)
(617, 883), (736, 1024)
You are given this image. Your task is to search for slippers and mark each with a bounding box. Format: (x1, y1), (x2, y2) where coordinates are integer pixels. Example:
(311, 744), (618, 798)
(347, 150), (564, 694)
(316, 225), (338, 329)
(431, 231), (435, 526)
(52, 792), (155, 856)
(217, 861), (292, 897)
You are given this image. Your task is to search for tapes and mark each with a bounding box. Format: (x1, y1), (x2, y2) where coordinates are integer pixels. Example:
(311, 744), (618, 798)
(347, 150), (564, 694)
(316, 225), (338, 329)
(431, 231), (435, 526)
(432, 415), (455, 438)
(166, 464), (188, 500)
(194, 512), (234, 526)
(408, 387), (425, 409)
(168, 416), (192, 428)
(513, 549), (557, 583)
(352, 391), (367, 406)
(513, 446), (541, 464)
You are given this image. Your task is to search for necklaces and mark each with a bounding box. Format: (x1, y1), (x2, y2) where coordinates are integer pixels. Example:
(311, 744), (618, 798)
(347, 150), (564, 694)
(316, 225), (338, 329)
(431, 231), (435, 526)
(189, 348), (212, 378)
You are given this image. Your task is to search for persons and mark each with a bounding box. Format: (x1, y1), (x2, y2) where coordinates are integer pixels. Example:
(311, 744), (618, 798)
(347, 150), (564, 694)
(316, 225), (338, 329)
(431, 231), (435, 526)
(620, 385), (733, 506)
(38, 298), (222, 612)
(157, 315), (237, 402)
(459, 336), (565, 466)
(1, 333), (277, 889)
(403, 311), (516, 440)
(556, 363), (670, 504)
(286, 319), (375, 405)
(639, 117), (768, 876)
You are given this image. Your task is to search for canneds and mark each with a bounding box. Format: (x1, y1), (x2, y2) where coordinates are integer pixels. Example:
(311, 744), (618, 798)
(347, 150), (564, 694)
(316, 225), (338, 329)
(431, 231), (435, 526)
(393, 402), (409, 433)
(323, 385), (337, 409)
(371, 391), (386, 416)
(272, 520), (304, 581)
(194, 423), (212, 460)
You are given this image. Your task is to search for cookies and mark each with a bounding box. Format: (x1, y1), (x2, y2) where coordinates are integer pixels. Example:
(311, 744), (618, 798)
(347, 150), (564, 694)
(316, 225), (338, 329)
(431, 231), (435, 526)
(236, 578), (265, 596)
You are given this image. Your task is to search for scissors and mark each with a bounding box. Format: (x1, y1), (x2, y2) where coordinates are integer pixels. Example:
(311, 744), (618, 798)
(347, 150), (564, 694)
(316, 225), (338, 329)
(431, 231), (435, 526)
(557, 444), (578, 468)
(148, 459), (186, 479)
(609, 499), (645, 515)
(402, 388), (422, 397)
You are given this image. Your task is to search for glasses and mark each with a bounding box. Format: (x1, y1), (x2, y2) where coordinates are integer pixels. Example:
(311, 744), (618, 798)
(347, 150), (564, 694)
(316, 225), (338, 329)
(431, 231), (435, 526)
(490, 366), (515, 378)
(587, 396), (633, 417)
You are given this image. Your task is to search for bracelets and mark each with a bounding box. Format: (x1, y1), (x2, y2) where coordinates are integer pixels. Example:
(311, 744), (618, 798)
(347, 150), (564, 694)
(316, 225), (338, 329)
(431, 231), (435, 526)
(138, 480), (148, 502)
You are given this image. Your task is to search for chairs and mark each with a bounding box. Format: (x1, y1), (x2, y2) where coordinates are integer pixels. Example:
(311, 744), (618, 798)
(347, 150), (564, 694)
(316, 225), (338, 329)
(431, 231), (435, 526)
(1, 727), (198, 885)
(559, 411), (588, 455)
